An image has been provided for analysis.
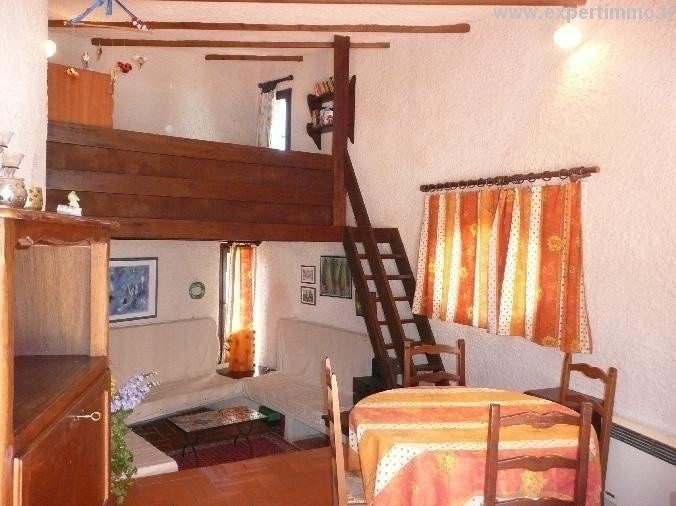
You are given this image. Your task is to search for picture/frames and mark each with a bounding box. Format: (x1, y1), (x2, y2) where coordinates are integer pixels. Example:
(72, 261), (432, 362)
(319, 254), (353, 299)
(107, 257), (159, 324)
(300, 286), (316, 305)
(300, 265), (315, 284)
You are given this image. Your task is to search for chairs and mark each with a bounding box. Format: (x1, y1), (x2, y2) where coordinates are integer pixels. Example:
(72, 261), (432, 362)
(555, 351), (619, 505)
(487, 403), (593, 503)
(404, 338), (469, 386)
(320, 357), (369, 504)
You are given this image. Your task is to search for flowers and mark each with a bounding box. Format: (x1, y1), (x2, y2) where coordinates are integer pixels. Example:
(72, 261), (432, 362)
(109, 369), (165, 506)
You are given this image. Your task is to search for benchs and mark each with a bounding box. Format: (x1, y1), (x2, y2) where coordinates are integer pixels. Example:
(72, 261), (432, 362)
(109, 421), (179, 481)
(108, 318), (245, 425)
(245, 319), (370, 436)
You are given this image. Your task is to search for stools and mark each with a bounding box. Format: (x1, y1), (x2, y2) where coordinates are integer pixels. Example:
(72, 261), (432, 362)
(525, 386), (604, 436)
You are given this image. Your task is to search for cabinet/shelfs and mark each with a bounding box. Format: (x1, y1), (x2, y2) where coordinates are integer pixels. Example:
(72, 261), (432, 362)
(2, 219), (122, 506)
